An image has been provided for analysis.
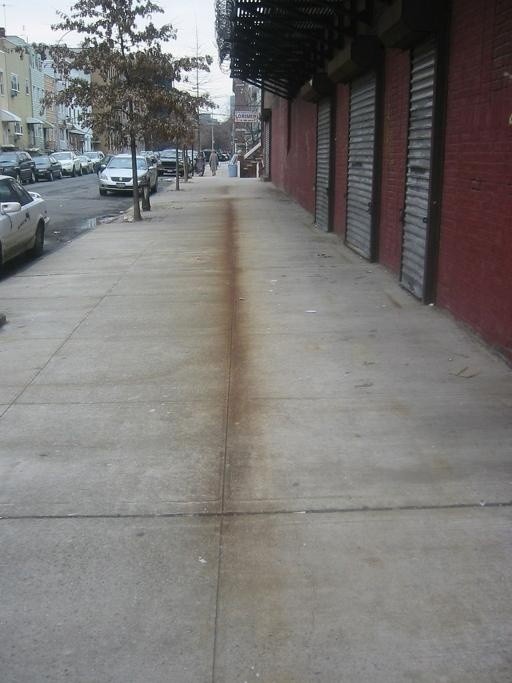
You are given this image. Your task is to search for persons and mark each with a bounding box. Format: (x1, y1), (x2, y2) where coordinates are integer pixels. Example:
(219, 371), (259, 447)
(194, 152), (208, 176)
(209, 150), (219, 176)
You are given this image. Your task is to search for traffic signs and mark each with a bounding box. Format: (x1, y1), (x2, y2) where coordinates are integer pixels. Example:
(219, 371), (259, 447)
(234, 110), (258, 122)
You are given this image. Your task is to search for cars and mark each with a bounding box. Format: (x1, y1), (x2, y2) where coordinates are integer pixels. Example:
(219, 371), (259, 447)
(0, 147), (231, 185)
(97, 152), (158, 195)
(0, 174), (47, 281)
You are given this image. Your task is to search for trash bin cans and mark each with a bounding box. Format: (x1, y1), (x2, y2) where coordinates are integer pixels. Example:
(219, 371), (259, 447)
(228, 164), (237, 177)
